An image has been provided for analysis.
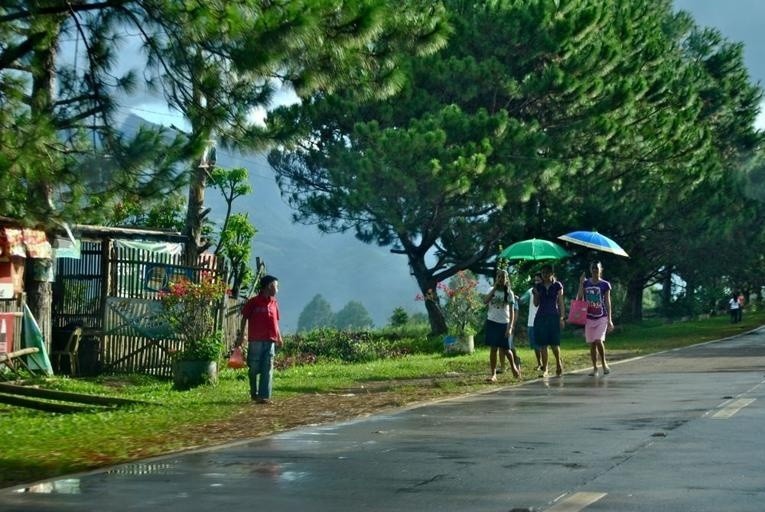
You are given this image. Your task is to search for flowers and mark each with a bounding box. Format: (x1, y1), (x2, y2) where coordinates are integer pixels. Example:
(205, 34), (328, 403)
(414, 271), (483, 334)
(157, 271), (228, 352)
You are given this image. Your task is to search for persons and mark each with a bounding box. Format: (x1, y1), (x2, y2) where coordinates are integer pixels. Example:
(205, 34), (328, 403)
(728, 293), (741, 324)
(482, 259), (615, 382)
(232, 274), (284, 405)
(736, 292), (746, 322)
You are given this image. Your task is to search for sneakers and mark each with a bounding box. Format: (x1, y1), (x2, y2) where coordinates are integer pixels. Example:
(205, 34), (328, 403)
(485, 358), (521, 382)
(250, 390), (270, 403)
(533, 364), (563, 378)
(589, 365), (610, 377)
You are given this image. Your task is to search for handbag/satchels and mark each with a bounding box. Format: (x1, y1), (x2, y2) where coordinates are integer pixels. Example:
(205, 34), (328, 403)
(569, 293), (589, 324)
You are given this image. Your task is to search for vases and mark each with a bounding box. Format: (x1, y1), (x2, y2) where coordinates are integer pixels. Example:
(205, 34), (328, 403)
(443, 332), (474, 356)
(166, 351), (222, 392)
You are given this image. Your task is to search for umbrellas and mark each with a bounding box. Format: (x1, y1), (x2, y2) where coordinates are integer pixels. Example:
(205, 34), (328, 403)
(496, 237), (573, 294)
(556, 230), (632, 273)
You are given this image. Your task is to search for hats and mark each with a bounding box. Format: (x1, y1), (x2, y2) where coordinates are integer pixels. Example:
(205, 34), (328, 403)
(261, 275), (277, 286)
